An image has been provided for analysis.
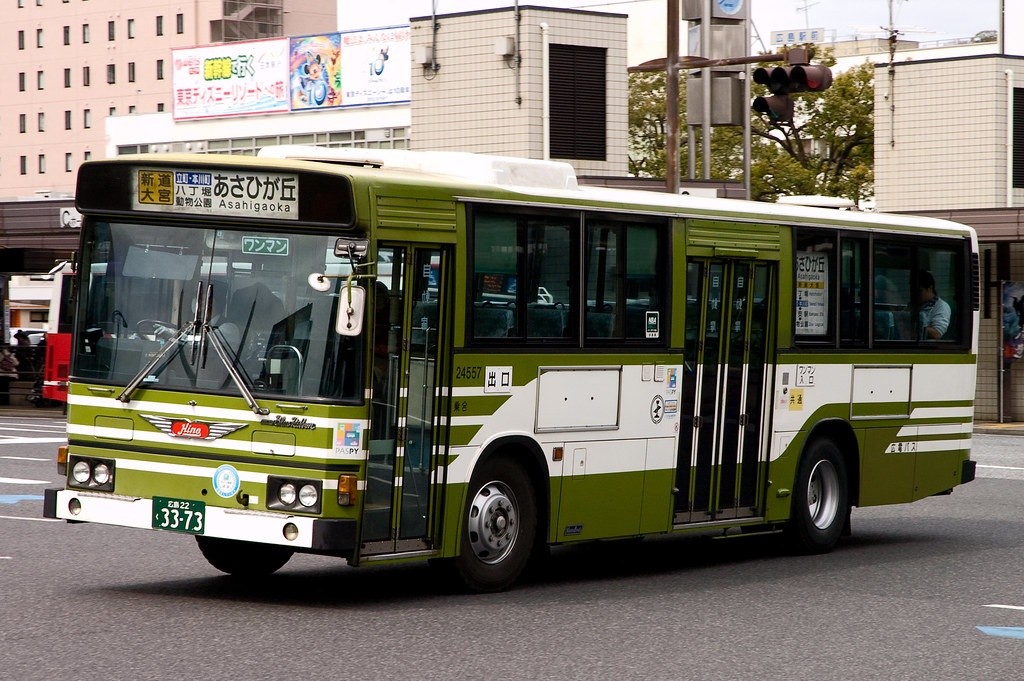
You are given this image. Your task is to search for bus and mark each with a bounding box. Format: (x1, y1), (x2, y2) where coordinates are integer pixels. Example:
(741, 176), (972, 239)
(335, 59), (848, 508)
(40, 262), (438, 404)
(41, 144), (983, 593)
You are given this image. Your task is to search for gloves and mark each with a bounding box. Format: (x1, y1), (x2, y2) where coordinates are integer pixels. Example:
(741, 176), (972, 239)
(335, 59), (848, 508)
(153, 323), (175, 336)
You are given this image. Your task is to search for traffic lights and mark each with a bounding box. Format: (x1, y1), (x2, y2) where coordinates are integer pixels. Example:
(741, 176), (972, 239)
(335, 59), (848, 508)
(749, 66), (833, 122)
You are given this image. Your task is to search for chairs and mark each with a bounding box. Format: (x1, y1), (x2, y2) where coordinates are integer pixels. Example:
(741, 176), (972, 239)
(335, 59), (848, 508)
(411, 301), (438, 352)
(586, 312), (616, 338)
(892, 312), (929, 341)
(853, 310), (895, 341)
(526, 308), (570, 338)
(627, 306), (650, 338)
(473, 308), (514, 338)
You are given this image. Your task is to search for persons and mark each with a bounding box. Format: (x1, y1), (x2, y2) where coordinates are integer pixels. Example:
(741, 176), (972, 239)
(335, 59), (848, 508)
(898, 269), (952, 341)
(153, 287), (243, 386)
(0, 332), (46, 408)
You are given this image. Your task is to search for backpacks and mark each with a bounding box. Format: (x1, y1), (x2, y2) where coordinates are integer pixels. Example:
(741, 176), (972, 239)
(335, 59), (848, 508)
(0, 350), (13, 371)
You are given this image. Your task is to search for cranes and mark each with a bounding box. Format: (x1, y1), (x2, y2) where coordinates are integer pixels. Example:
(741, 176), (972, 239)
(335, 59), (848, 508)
(797, 0), (822, 28)
